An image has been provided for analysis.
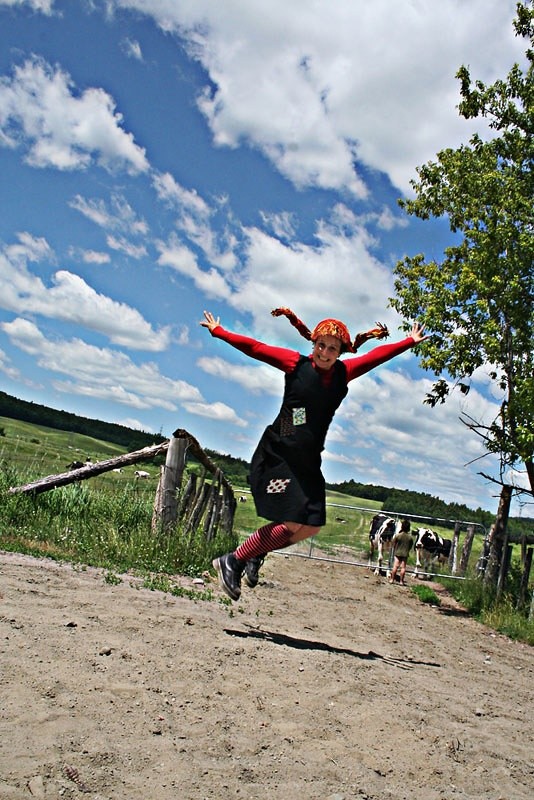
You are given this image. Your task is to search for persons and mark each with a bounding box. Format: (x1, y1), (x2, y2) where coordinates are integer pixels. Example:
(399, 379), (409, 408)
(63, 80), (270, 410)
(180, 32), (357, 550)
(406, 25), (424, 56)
(199, 307), (431, 602)
(388, 520), (414, 586)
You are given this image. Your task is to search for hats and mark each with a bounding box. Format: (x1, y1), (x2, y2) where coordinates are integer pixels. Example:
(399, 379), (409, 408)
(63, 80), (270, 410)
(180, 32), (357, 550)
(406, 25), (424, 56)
(270, 307), (390, 353)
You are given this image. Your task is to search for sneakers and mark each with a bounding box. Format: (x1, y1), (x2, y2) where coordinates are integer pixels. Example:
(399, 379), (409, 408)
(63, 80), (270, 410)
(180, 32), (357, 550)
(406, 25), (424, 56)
(213, 553), (246, 601)
(243, 557), (264, 587)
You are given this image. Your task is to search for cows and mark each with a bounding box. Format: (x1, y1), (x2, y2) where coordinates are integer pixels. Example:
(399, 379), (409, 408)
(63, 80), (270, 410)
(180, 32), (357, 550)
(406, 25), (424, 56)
(367, 512), (403, 579)
(65, 456), (150, 480)
(411, 527), (452, 580)
(238, 496), (247, 502)
